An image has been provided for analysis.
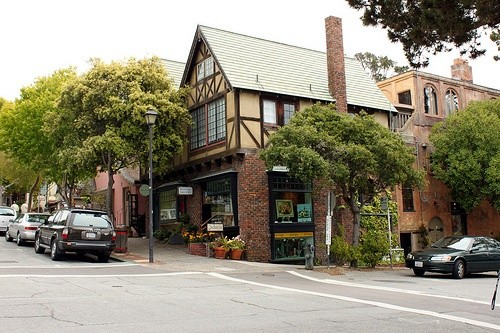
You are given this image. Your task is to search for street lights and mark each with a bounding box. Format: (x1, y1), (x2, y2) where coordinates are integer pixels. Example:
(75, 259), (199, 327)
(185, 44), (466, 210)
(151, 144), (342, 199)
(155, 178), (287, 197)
(144, 107), (158, 263)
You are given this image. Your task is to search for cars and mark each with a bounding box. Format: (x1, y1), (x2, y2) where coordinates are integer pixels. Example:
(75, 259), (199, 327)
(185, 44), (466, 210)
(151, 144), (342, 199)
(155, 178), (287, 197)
(5, 212), (51, 246)
(0, 205), (17, 234)
(405, 235), (500, 279)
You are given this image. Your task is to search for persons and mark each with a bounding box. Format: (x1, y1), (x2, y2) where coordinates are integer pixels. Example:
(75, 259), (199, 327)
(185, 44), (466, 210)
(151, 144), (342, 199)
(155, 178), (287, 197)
(11, 201), (19, 212)
(21, 201), (29, 213)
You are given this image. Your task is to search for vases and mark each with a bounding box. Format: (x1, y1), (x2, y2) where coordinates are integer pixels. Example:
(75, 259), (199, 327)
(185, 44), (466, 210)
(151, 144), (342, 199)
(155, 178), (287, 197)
(212, 247), (228, 259)
(229, 248), (243, 259)
(188, 241), (211, 256)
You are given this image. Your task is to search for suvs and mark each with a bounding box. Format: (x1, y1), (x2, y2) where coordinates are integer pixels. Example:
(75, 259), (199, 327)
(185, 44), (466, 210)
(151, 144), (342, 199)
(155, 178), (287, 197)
(34, 207), (117, 263)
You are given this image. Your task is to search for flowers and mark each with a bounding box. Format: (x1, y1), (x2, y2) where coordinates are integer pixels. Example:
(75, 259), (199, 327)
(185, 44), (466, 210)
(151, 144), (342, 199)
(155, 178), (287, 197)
(209, 232), (231, 250)
(229, 236), (246, 251)
(188, 231), (219, 243)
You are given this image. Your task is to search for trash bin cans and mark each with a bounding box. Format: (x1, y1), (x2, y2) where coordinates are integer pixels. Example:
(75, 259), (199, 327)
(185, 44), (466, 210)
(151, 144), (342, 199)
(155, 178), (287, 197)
(114, 224), (129, 253)
